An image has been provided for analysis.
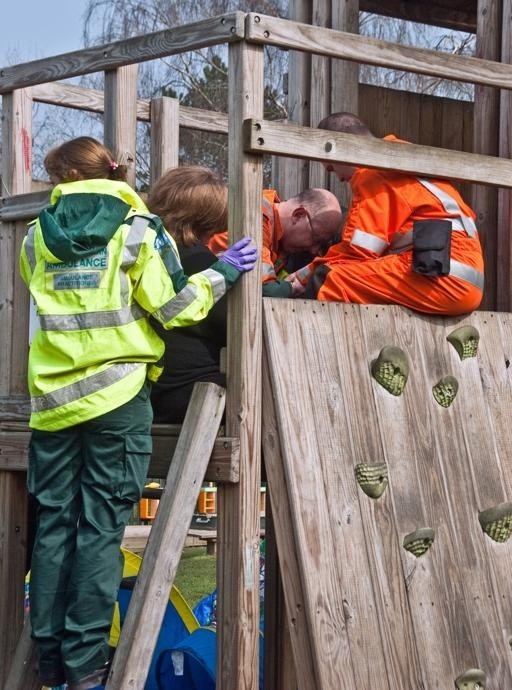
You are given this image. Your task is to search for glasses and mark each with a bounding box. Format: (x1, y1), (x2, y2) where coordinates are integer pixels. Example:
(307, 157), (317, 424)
(304, 210), (334, 246)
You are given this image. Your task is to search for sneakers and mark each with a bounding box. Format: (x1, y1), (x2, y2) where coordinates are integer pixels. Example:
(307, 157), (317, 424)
(40, 645), (116, 690)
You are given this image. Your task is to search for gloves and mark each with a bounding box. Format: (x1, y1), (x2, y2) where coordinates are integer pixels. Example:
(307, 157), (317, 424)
(217, 236), (257, 272)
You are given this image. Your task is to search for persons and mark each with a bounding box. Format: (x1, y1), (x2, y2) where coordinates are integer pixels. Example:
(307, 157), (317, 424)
(284, 110), (487, 319)
(143, 164), (295, 424)
(207, 187), (344, 298)
(17, 134), (260, 690)
